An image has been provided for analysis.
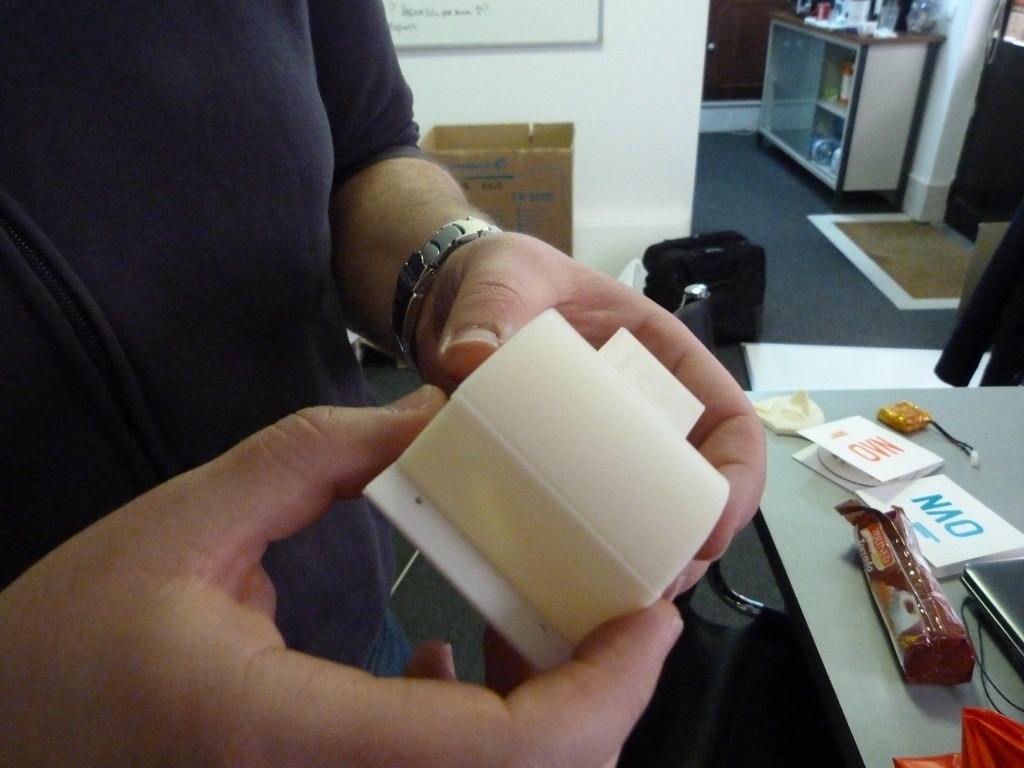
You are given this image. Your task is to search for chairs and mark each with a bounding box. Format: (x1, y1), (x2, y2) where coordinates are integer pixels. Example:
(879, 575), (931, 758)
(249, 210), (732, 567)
(620, 284), (864, 767)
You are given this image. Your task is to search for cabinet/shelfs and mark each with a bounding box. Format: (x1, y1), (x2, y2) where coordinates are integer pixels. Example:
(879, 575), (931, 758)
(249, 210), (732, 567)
(756, 11), (944, 211)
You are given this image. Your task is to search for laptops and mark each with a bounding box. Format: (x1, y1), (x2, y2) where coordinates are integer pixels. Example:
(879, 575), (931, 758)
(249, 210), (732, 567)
(960, 556), (1024, 672)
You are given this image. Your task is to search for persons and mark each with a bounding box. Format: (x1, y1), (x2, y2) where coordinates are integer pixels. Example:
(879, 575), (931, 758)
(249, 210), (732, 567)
(0, 0), (769, 768)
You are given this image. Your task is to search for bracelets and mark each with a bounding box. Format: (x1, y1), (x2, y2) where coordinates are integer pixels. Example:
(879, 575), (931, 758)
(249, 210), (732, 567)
(391, 216), (503, 376)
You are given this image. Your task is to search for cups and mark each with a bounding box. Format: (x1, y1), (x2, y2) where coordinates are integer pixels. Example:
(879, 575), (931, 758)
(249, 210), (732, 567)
(878, 7), (899, 34)
(855, 22), (877, 37)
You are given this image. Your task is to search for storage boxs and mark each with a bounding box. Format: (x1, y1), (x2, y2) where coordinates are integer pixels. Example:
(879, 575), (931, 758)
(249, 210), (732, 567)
(417, 120), (575, 260)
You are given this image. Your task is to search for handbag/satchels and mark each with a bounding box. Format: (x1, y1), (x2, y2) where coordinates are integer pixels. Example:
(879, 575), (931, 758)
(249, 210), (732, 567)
(643, 230), (767, 348)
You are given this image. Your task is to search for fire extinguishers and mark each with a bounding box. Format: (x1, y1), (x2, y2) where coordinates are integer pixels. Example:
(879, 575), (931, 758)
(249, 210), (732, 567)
(840, 56), (855, 106)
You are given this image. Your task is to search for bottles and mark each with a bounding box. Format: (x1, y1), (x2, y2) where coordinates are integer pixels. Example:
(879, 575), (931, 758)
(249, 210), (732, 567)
(837, 59), (855, 105)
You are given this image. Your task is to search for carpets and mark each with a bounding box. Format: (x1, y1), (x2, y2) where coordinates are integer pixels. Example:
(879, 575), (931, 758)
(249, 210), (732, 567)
(806, 212), (975, 312)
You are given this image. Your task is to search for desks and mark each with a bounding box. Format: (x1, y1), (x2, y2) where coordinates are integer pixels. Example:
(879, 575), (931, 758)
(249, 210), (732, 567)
(742, 386), (1023, 768)
